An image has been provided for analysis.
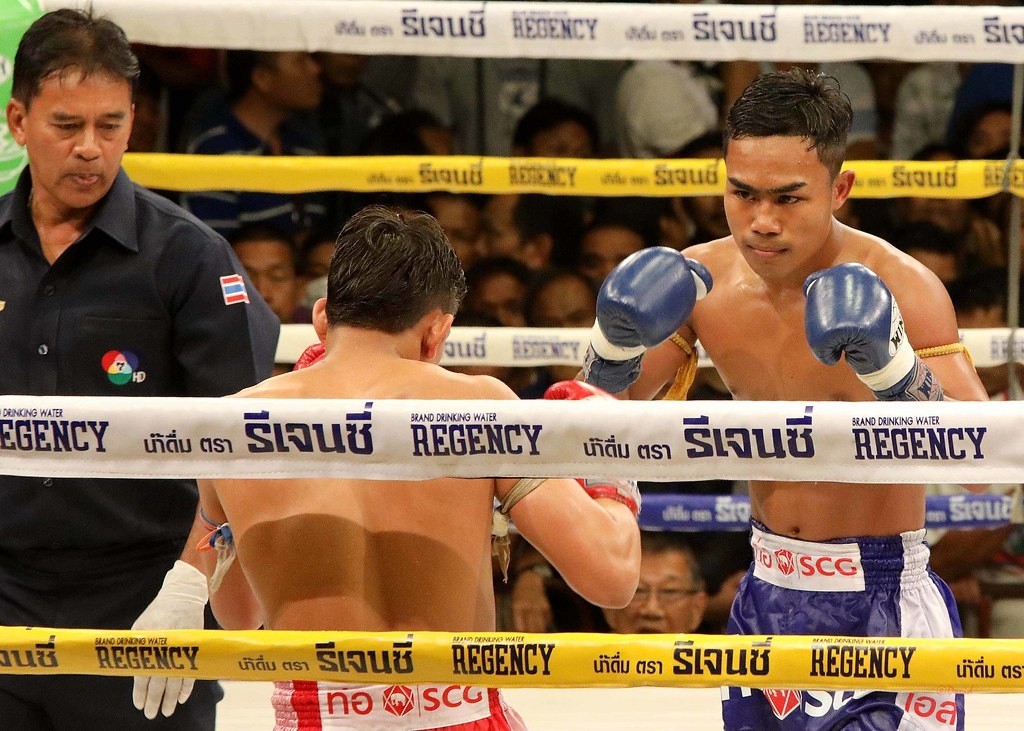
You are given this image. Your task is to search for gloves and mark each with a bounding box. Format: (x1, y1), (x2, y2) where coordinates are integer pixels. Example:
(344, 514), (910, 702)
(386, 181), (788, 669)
(544, 380), (642, 518)
(581, 246), (714, 393)
(294, 342), (325, 372)
(127, 560), (211, 721)
(803, 261), (919, 396)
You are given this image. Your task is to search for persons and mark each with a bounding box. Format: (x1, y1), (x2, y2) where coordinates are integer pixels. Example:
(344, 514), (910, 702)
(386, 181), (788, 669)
(129, 44), (764, 633)
(0, 10), (280, 731)
(723, 61), (1023, 638)
(579, 70), (992, 730)
(197, 208), (641, 730)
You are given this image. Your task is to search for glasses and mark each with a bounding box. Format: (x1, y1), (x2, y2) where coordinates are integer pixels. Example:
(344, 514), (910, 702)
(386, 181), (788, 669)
(634, 577), (699, 603)
